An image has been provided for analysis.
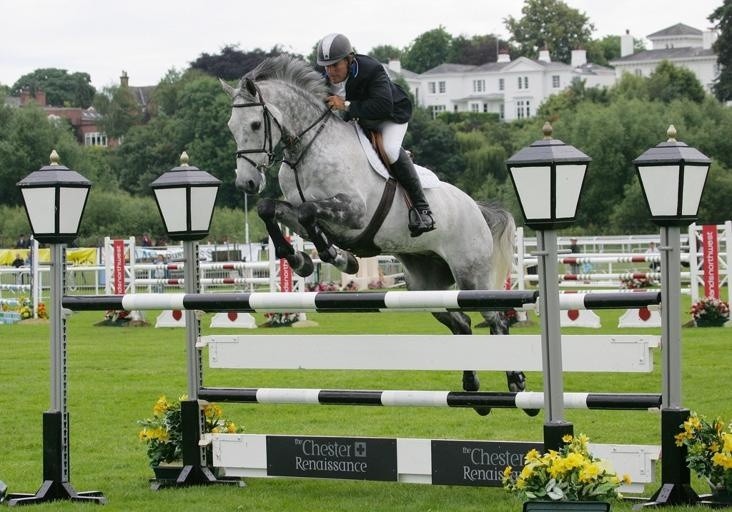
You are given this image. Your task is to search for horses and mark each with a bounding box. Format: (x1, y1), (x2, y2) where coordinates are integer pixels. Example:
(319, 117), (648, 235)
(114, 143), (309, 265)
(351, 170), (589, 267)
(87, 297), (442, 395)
(217, 54), (540, 417)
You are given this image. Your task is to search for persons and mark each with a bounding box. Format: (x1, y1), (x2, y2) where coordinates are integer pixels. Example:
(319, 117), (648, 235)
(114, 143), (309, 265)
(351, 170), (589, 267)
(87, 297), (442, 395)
(313, 32), (438, 232)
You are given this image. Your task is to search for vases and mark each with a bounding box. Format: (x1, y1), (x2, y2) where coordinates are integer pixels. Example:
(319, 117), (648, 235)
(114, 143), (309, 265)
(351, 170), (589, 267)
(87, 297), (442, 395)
(150, 466), (226, 480)
(695, 318), (730, 327)
(704, 476), (732, 509)
(522, 500), (611, 512)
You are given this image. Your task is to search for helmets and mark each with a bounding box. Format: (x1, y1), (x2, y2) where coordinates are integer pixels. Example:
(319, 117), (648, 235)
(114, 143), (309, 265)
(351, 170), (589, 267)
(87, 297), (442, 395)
(317, 33), (353, 67)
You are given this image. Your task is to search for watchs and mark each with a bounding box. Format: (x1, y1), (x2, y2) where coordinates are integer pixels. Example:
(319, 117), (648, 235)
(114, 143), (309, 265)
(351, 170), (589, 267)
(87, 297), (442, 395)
(343, 100), (351, 111)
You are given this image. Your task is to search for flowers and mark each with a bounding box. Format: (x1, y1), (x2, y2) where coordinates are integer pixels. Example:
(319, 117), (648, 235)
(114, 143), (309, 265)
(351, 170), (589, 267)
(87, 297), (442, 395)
(501, 432), (632, 504)
(674, 410), (732, 491)
(136, 393), (248, 467)
(689, 296), (730, 321)
(0, 294), (50, 321)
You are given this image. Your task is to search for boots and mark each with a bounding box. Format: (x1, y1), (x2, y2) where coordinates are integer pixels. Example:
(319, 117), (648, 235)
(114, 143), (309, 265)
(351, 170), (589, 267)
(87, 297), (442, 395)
(388, 148), (436, 231)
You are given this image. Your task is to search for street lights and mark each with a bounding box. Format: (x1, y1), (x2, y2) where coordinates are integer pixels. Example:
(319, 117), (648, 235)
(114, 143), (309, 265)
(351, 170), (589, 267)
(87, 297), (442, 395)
(148, 149), (247, 491)
(624, 125), (731, 511)
(506, 122), (594, 501)
(6, 149), (102, 504)
